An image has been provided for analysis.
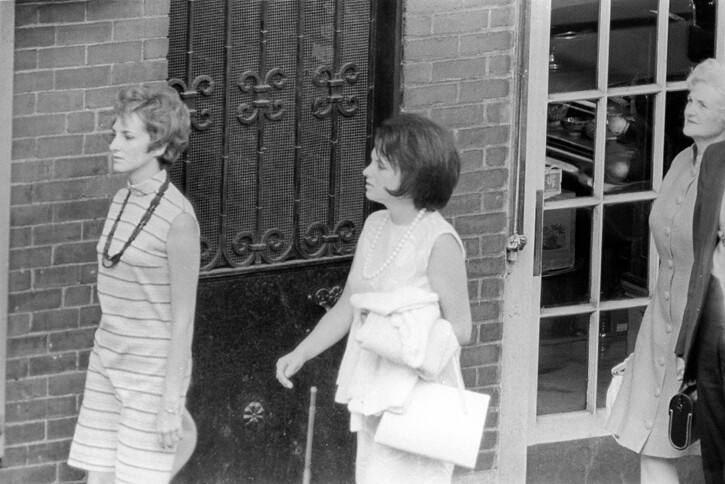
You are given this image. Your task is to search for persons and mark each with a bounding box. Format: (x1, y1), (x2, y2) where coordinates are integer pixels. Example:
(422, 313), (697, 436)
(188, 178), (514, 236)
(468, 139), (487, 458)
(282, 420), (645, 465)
(67, 82), (201, 484)
(606, 55), (725, 484)
(275, 114), (472, 484)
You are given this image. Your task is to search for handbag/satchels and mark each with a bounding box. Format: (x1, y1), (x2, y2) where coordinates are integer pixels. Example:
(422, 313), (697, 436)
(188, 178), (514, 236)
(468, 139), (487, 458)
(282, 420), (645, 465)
(668, 380), (699, 450)
(373, 378), (491, 469)
(169, 408), (197, 482)
(606, 353), (632, 422)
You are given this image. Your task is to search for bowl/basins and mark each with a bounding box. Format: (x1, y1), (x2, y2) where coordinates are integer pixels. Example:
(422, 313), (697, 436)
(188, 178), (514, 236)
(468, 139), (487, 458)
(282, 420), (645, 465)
(560, 116), (589, 136)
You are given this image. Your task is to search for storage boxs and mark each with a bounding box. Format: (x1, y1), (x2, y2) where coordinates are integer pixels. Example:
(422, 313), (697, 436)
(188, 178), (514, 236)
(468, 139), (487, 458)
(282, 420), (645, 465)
(542, 193), (576, 271)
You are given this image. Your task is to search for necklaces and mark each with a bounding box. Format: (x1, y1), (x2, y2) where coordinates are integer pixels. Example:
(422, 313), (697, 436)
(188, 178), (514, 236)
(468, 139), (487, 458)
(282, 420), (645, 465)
(101, 170), (171, 268)
(361, 207), (426, 280)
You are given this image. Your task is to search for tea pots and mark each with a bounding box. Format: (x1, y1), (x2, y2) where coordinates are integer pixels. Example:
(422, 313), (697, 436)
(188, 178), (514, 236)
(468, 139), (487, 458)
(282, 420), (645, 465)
(606, 107), (635, 141)
